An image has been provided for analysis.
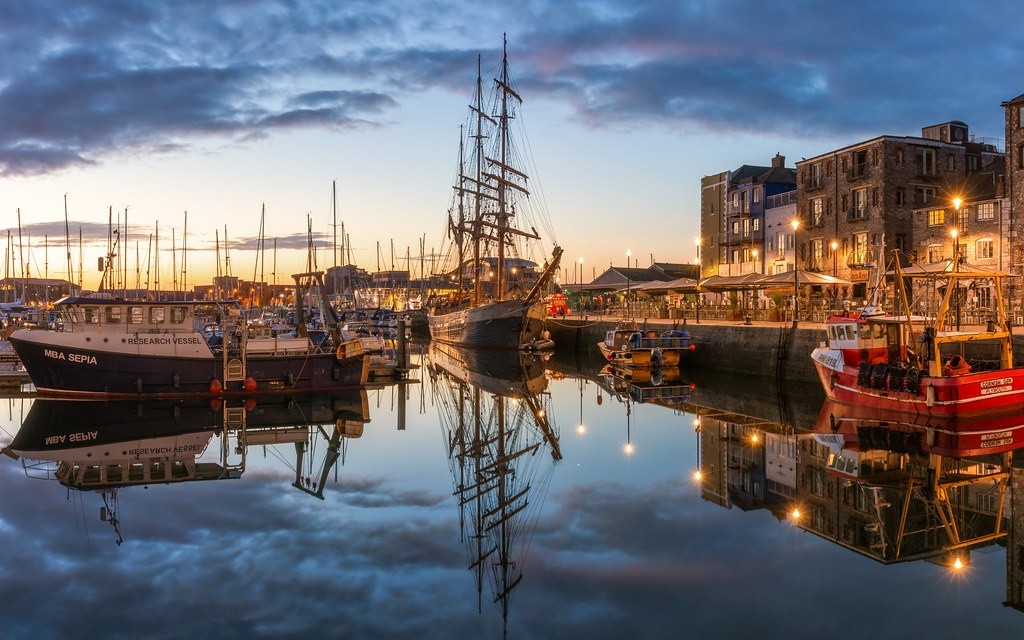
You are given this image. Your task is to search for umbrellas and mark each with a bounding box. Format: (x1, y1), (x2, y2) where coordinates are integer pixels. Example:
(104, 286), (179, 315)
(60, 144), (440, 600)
(756, 269), (853, 285)
(880, 258), (1018, 277)
(618, 273), (773, 289)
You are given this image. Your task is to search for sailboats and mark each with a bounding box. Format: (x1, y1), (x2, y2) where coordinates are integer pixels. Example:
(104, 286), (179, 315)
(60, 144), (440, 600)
(425, 342), (562, 640)
(1, 181), (480, 387)
(432, 27), (549, 353)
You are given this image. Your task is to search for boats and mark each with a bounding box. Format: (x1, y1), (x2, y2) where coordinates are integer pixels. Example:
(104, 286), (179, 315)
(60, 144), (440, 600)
(813, 398), (1021, 491)
(3, 394), (363, 505)
(810, 241), (1024, 419)
(597, 320), (706, 372)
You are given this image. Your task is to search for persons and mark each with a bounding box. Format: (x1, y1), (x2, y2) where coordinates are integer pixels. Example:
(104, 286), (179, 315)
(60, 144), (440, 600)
(556, 294), (854, 312)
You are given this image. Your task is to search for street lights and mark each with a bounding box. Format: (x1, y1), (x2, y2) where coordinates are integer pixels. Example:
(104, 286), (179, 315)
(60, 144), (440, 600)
(749, 248), (758, 277)
(791, 218), (803, 321)
(626, 250), (632, 317)
(830, 241), (838, 280)
(951, 187), (964, 338)
(567, 375), (584, 434)
(578, 258), (585, 315)
(689, 412), (702, 482)
(783, 436), (807, 523)
(693, 238), (701, 323)
(620, 395), (636, 453)
